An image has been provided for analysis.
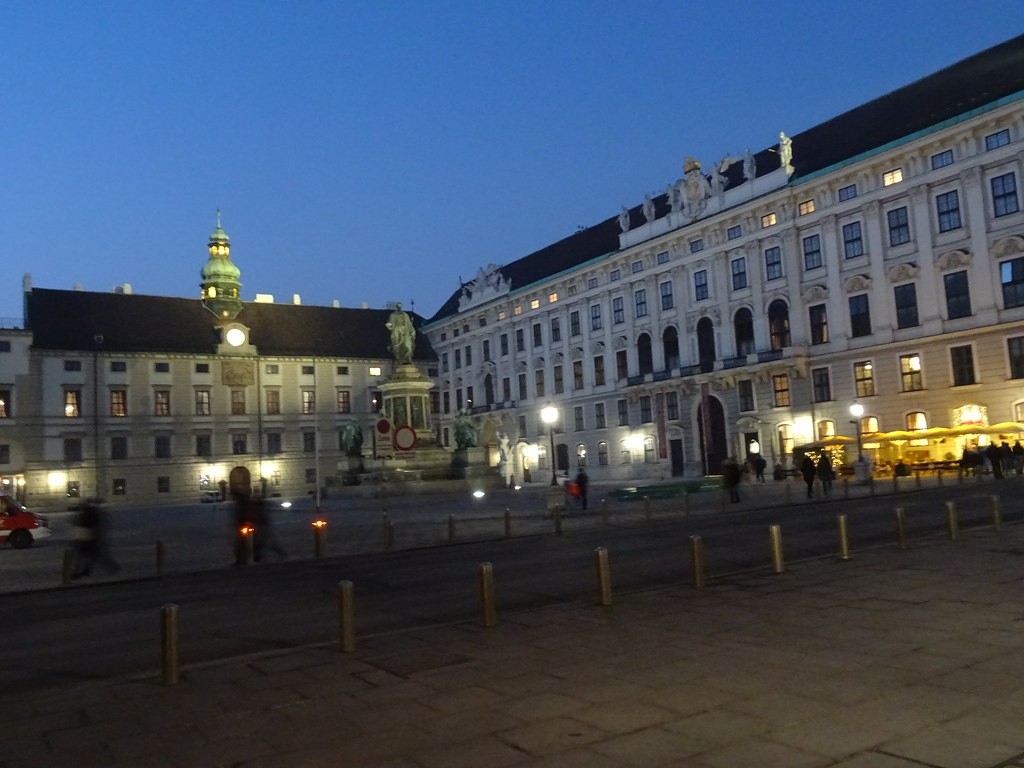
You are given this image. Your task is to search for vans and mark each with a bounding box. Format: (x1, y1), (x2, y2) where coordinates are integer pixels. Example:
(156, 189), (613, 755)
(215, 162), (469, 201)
(0, 492), (54, 550)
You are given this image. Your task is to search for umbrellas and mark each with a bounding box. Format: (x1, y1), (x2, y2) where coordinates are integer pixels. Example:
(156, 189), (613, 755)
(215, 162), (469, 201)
(816, 422), (1024, 466)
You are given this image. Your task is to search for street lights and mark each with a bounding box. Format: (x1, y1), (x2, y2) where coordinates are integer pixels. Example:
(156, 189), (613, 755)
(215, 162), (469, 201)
(541, 406), (561, 487)
(848, 402), (868, 463)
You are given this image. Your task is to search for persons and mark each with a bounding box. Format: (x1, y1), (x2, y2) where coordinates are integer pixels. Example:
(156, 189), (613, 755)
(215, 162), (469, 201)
(496, 433), (514, 461)
(642, 195), (653, 220)
(344, 419), (363, 454)
(385, 303), (416, 365)
(874, 457), (911, 477)
(71, 498), (119, 578)
(574, 468), (589, 509)
(231, 485), (283, 563)
(801, 453), (836, 498)
(778, 132), (792, 175)
(665, 158), (726, 215)
(725, 457), (742, 503)
(455, 409), (479, 447)
(616, 209), (630, 232)
(738, 148), (755, 180)
(960, 440), (1024, 477)
(748, 453), (767, 482)
(478, 264), (504, 292)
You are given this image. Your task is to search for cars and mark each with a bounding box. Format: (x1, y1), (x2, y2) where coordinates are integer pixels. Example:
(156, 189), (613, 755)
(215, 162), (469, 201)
(201, 491), (222, 504)
